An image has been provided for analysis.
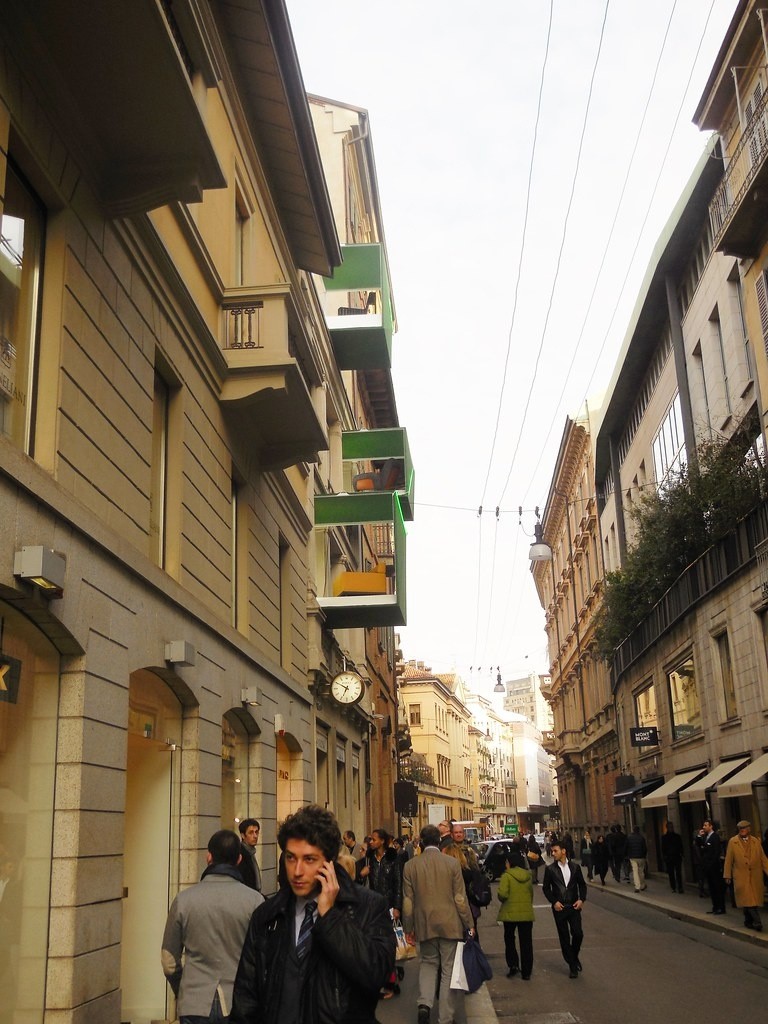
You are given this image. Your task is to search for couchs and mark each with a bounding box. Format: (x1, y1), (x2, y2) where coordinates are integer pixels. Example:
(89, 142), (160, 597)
(332, 561), (387, 597)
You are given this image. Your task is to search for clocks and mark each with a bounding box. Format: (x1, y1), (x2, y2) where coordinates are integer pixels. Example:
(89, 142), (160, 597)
(330, 671), (365, 707)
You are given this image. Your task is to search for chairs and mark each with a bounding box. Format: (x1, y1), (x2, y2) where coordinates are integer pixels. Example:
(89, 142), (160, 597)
(353, 458), (402, 492)
(337, 291), (377, 315)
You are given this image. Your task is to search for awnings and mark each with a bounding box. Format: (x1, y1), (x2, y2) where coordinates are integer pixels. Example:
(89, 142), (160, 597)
(613, 753), (768, 808)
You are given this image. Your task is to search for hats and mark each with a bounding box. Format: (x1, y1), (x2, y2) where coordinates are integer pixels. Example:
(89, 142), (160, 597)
(738, 821), (750, 827)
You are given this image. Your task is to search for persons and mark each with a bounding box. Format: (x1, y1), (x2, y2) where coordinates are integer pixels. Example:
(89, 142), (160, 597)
(579, 824), (648, 893)
(162, 804), (492, 1024)
(691, 819), (768, 930)
(660, 821), (684, 894)
(497, 829), (587, 980)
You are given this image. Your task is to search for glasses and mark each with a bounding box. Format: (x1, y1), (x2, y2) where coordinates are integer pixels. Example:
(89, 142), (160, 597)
(439, 823), (447, 827)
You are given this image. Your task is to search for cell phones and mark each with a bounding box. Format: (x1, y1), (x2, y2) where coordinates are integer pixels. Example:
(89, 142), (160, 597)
(316, 857), (335, 893)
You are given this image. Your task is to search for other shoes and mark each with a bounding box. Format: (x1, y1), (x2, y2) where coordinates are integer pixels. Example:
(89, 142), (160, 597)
(378, 983), (401, 999)
(506, 964), (519, 977)
(570, 970), (578, 978)
(641, 884), (647, 891)
(537, 880), (539, 882)
(417, 1004), (430, 1024)
(522, 973), (530, 980)
(714, 910), (726, 914)
(533, 882), (536, 884)
(706, 910), (715, 913)
(635, 889), (639, 892)
(576, 958), (582, 971)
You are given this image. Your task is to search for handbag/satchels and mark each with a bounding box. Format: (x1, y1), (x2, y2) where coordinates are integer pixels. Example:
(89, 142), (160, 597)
(527, 850), (539, 862)
(451, 930), (492, 995)
(392, 917), (417, 961)
(468, 880), (491, 907)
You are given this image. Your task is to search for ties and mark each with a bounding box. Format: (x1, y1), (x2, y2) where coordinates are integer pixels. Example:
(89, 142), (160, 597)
(293, 902), (315, 962)
